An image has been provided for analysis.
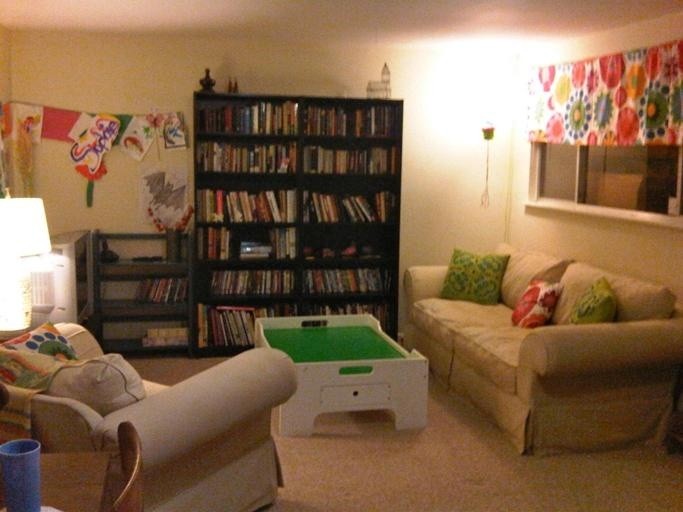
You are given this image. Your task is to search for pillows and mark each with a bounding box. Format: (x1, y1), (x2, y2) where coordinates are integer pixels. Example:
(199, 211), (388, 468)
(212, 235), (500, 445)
(439, 246), (673, 330)
(3, 319), (79, 361)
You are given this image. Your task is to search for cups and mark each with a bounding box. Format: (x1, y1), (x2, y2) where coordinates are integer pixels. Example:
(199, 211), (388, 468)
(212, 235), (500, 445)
(0, 439), (41, 512)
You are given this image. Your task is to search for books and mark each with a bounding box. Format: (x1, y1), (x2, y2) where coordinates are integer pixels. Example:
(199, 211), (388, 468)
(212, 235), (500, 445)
(300, 303), (390, 330)
(143, 327), (188, 346)
(138, 278), (188, 303)
(197, 141), (297, 173)
(195, 226), (295, 260)
(299, 188), (396, 222)
(302, 104), (393, 137)
(302, 144), (396, 174)
(305, 270), (392, 293)
(210, 269), (293, 295)
(197, 303), (297, 346)
(196, 188), (297, 222)
(197, 101), (298, 135)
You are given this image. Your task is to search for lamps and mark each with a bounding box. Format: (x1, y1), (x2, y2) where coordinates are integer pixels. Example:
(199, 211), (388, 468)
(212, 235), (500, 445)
(481, 128), (494, 208)
(1, 197), (52, 330)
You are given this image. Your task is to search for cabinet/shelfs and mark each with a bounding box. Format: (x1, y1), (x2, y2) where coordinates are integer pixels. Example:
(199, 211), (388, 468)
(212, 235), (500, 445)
(193, 92), (403, 358)
(85, 230), (194, 357)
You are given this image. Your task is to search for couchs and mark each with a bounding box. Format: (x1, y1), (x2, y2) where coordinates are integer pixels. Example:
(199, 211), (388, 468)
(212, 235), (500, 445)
(403, 245), (682, 456)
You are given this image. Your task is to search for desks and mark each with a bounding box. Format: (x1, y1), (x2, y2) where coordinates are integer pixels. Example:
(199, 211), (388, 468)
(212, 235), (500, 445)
(0, 452), (108, 511)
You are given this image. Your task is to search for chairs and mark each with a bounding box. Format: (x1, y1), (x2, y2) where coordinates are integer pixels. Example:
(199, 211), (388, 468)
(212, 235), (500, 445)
(0, 323), (298, 512)
(109, 422), (143, 512)
